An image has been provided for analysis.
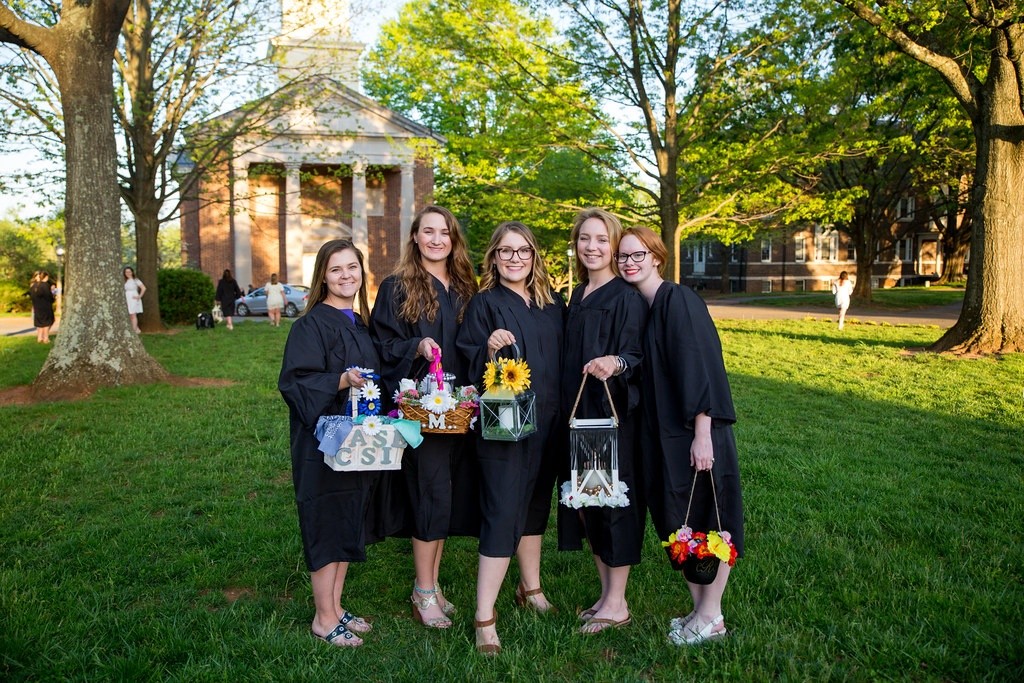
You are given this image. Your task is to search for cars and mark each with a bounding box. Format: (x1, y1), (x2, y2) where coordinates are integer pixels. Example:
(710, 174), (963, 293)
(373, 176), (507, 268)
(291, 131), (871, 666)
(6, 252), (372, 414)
(895, 274), (940, 289)
(236, 285), (313, 318)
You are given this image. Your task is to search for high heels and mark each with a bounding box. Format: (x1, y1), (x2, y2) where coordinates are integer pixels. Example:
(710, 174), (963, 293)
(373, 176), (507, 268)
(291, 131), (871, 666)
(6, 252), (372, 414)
(433, 581), (459, 616)
(411, 579), (452, 629)
(514, 580), (559, 617)
(472, 606), (503, 655)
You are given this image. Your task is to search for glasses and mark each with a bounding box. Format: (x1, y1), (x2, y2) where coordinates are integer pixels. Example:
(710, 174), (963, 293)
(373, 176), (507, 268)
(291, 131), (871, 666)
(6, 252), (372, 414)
(494, 245), (535, 261)
(612, 250), (652, 263)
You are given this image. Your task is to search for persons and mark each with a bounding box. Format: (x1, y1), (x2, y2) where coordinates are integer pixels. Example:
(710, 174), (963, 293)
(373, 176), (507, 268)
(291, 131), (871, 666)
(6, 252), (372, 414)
(832, 271), (853, 328)
(123, 267), (147, 334)
(277, 239), (385, 647)
(264, 274), (288, 327)
(368, 206), (478, 628)
(240, 284), (259, 296)
(215, 269), (241, 330)
(619, 226), (745, 647)
(556, 207), (651, 636)
(23, 270), (57, 343)
(454, 221), (567, 660)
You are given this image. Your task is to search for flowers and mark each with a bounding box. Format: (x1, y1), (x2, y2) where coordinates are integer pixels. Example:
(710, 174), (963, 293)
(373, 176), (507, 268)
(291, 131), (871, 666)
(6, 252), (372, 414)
(662, 525), (738, 566)
(347, 367), (382, 435)
(483, 357), (532, 392)
(559, 480), (632, 509)
(389, 385), (481, 421)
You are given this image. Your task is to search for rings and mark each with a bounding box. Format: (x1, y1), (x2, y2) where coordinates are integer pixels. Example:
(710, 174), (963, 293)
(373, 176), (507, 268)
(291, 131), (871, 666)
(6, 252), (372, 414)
(713, 458), (715, 462)
(497, 344), (498, 346)
(603, 379), (607, 381)
(587, 362), (591, 365)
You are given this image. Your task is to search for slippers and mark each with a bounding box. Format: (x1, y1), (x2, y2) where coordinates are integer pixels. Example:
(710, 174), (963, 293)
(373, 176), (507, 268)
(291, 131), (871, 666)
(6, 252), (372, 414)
(580, 611), (632, 634)
(337, 610), (373, 632)
(577, 603), (598, 621)
(313, 620), (364, 649)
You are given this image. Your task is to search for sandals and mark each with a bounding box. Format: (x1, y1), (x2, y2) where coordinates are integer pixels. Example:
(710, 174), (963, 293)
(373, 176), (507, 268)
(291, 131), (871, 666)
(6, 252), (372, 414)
(670, 607), (696, 630)
(667, 612), (727, 644)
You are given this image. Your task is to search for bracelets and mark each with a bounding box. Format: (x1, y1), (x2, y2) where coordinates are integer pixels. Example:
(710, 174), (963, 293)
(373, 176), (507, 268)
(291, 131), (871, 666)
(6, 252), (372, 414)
(418, 350), (423, 355)
(608, 354), (627, 376)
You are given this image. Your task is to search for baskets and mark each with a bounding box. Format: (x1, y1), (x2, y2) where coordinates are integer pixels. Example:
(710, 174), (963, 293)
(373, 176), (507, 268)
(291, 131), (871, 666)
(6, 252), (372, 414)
(398, 346), (471, 434)
(322, 377), (409, 472)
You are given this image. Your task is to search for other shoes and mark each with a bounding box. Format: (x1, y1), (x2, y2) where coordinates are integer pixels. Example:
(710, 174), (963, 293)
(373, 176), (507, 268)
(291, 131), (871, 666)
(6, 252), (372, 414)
(270, 320), (281, 328)
(226, 323), (234, 330)
